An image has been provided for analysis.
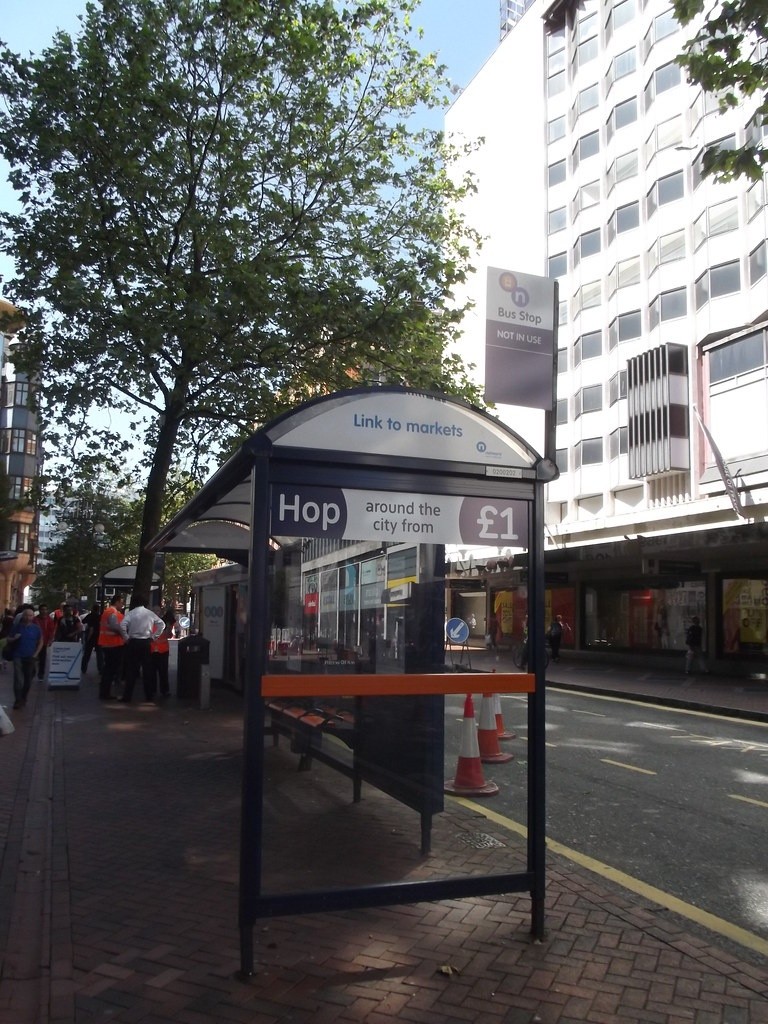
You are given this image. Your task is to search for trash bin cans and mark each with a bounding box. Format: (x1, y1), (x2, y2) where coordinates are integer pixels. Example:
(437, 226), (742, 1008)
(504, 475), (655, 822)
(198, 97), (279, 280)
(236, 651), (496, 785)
(168, 635), (211, 709)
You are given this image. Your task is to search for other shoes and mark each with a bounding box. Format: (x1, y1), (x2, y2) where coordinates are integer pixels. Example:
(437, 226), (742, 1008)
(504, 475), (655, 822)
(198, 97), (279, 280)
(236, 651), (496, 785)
(145, 698), (151, 702)
(164, 693), (172, 698)
(39, 678), (43, 682)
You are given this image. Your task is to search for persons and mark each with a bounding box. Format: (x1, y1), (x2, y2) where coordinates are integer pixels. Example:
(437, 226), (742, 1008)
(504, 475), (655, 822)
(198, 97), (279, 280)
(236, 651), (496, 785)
(0, 595), (176, 710)
(485, 612), (504, 652)
(685, 616), (708, 675)
(469, 614), (477, 637)
(658, 602), (670, 649)
(545, 615), (564, 663)
(519, 615), (528, 670)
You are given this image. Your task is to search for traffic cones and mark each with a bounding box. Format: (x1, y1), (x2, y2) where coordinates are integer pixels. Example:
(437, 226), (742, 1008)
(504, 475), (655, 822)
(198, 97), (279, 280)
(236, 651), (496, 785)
(475, 692), (513, 764)
(441, 693), (499, 797)
(489, 690), (516, 741)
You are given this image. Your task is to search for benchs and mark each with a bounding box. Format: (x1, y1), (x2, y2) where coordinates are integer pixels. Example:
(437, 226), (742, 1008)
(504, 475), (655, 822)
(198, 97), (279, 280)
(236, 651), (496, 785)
(263, 697), (361, 803)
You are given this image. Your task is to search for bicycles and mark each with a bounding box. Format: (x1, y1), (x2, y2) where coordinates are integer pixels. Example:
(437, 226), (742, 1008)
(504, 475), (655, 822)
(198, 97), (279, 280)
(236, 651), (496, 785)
(514, 632), (552, 669)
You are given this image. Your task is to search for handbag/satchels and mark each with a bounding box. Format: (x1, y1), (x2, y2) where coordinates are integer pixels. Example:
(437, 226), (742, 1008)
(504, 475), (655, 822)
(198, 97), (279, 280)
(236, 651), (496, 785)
(3, 637), (22, 662)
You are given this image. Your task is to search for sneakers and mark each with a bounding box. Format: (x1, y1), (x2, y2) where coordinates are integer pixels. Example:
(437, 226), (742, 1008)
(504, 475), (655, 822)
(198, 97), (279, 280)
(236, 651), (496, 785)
(13, 701), (23, 710)
(22, 700), (26, 706)
(100, 696), (123, 702)
(116, 699), (130, 703)
